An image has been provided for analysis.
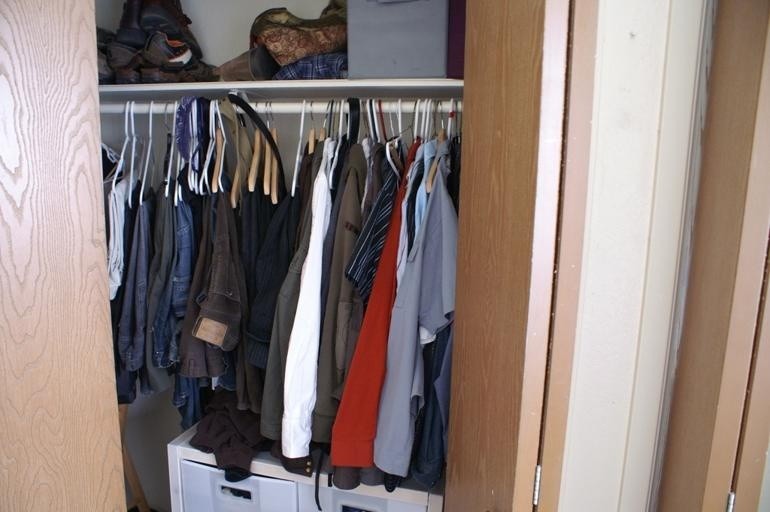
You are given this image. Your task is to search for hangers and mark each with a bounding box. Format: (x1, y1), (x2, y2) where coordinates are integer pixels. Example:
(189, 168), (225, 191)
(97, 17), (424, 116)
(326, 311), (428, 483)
(111, 100), (279, 210)
(291, 98), (454, 199)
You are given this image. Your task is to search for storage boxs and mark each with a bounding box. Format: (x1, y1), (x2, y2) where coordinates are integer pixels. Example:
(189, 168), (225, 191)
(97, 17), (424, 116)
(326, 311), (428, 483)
(347, 0), (449, 80)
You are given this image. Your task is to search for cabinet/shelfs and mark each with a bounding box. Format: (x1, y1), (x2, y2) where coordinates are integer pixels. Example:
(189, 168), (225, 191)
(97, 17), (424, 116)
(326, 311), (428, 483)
(167, 419), (444, 512)
